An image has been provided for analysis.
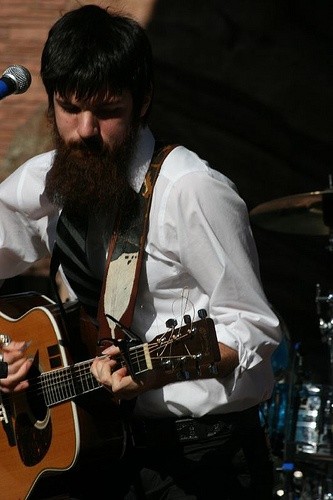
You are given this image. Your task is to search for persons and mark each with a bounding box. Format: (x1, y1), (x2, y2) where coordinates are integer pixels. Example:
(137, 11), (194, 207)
(0, 3), (291, 500)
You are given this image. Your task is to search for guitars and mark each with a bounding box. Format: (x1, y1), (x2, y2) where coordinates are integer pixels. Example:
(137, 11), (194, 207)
(0, 289), (222, 500)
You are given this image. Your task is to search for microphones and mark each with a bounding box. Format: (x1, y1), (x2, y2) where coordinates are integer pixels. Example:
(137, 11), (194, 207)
(0, 64), (32, 100)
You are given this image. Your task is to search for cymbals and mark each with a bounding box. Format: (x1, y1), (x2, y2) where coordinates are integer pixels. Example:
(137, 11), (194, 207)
(249, 189), (333, 236)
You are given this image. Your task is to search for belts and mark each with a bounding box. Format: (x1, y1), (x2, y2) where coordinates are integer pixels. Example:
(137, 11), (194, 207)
(177, 403), (271, 447)
